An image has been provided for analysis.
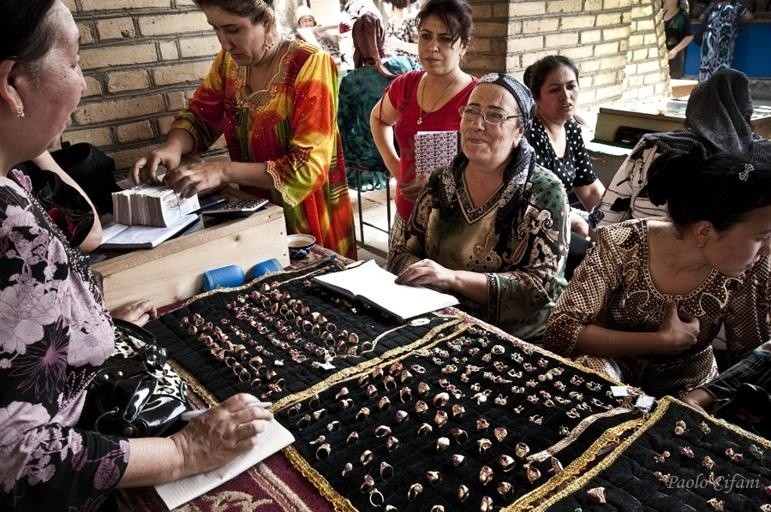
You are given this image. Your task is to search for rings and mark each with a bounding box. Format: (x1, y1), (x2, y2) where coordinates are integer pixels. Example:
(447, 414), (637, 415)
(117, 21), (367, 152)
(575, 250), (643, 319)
(175, 271), (771, 512)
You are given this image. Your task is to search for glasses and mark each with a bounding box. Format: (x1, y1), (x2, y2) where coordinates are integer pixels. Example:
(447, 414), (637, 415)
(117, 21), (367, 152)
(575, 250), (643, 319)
(458, 106), (522, 125)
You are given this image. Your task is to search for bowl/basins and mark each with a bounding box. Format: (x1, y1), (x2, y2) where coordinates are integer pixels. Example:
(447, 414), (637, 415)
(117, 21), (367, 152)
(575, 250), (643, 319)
(285, 233), (316, 258)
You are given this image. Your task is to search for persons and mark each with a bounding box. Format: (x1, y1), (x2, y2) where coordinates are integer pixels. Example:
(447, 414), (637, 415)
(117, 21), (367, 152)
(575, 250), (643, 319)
(130, 2), (769, 443)
(0, 1), (273, 512)
(8, 151), (103, 253)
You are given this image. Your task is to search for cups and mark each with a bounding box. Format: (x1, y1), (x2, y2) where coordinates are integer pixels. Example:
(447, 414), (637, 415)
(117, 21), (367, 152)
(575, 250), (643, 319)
(249, 257), (284, 280)
(202, 264), (244, 292)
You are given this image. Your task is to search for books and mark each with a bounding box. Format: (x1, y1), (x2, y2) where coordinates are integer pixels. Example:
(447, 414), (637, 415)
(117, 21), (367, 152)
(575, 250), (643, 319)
(100, 212), (199, 248)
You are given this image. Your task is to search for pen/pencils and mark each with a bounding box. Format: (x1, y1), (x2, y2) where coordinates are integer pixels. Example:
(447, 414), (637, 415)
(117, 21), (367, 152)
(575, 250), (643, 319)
(181, 402), (272, 421)
(201, 198), (226, 209)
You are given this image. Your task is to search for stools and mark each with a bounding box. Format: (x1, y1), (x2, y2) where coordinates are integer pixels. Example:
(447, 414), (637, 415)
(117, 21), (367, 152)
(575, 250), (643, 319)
(349, 169), (393, 258)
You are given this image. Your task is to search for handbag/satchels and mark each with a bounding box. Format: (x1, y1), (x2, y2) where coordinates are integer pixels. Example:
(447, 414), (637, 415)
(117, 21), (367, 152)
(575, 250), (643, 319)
(50, 141), (123, 215)
(76, 317), (193, 437)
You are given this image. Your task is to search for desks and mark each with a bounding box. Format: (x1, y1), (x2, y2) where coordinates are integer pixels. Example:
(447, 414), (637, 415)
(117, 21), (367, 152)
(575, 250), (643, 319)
(594, 99), (770, 144)
(118, 245), (771, 512)
